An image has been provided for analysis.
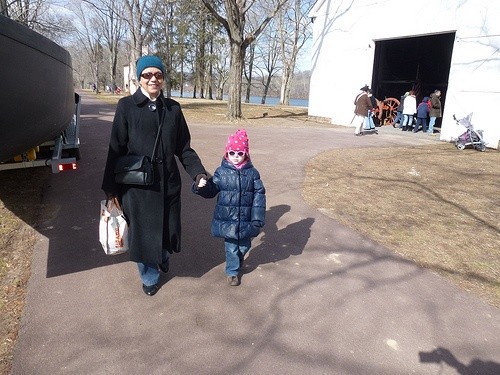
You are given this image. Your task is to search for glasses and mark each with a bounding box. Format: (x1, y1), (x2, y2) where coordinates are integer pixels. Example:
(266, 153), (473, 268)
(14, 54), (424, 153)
(227, 150), (246, 157)
(140, 71), (164, 79)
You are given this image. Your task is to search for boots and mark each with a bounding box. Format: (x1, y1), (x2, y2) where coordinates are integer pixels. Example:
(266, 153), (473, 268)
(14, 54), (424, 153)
(402, 125), (412, 131)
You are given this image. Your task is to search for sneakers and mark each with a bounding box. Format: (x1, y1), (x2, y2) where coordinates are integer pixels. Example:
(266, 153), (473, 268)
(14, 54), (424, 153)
(227, 274), (240, 286)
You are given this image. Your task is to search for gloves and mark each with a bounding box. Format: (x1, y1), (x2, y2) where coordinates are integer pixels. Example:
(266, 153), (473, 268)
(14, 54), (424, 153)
(193, 174), (208, 191)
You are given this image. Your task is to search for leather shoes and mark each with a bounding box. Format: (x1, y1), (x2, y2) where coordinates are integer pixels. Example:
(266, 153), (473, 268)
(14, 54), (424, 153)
(159, 260), (169, 273)
(142, 283), (158, 296)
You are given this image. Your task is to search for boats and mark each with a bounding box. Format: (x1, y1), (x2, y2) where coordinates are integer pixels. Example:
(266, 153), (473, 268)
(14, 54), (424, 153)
(0, 13), (75, 162)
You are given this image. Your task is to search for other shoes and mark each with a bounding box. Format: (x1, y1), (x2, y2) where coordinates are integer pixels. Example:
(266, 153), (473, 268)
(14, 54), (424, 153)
(412, 129), (417, 133)
(354, 132), (366, 136)
(391, 122), (395, 128)
(423, 130), (428, 133)
(364, 128), (375, 130)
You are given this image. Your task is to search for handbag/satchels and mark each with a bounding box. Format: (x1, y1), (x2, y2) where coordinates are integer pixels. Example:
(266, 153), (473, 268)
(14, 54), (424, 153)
(245, 226), (261, 239)
(112, 97), (167, 186)
(99, 197), (129, 256)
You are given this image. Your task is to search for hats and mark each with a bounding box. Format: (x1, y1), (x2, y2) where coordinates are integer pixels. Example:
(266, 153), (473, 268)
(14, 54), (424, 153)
(135, 55), (165, 81)
(368, 89), (375, 95)
(404, 92), (410, 97)
(225, 129), (249, 157)
(422, 97), (428, 102)
(361, 86), (369, 91)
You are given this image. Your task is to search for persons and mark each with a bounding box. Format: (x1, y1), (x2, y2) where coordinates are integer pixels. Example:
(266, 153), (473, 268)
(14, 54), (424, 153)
(101, 56), (207, 297)
(392, 90), (442, 134)
(354, 85), (378, 135)
(112, 84), (121, 95)
(92, 84), (96, 92)
(197, 128), (266, 286)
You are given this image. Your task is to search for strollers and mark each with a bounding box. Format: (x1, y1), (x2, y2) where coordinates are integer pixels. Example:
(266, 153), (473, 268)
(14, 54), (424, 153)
(452, 113), (487, 152)
